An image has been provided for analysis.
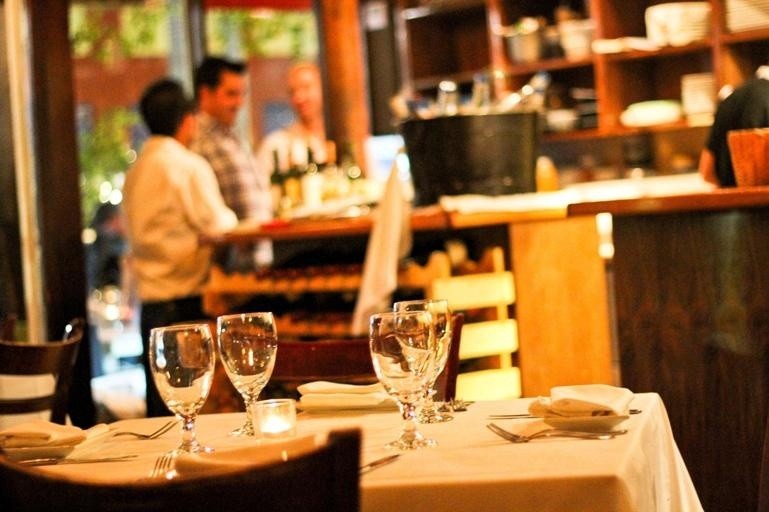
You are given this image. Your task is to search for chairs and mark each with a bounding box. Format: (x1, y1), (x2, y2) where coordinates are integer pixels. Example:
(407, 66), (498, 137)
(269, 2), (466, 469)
(0, 318), (87, 426)
(0, 424), (364, 512)
(267, 308), (468, 417)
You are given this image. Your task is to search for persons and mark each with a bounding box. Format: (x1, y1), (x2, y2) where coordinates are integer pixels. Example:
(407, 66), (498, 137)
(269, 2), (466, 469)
(84, 202), (125, 377)
(124, 79), (237, 418)
(700, 42), (769, 189)
(255, 61), (337, 271)
(186, 55), (274, 318)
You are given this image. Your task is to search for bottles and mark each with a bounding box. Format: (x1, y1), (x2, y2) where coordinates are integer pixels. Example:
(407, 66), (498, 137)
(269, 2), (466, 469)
(268, 148), (284, 211)
(284, 144), (301, 208)
(341, 136), (360, 184)
(303, 140), (319, 179)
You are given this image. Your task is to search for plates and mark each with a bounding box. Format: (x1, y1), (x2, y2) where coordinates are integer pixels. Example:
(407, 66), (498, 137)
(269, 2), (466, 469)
(300, 391), (382, 412)
(3, 446), (75, 460)
(544, 415), (630, 429)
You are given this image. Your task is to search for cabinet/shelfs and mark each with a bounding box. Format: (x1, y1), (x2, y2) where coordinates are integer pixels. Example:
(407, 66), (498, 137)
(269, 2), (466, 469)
(385, 0), (768, 142)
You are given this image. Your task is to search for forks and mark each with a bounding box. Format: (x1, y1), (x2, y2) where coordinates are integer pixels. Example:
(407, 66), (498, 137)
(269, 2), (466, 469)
(110, 419), (179, 440)
(487, 423), (628, 443)
(152, 449), (175, 476)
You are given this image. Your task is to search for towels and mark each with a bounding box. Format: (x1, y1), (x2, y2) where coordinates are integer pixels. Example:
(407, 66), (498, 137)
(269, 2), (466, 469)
(352, 429), (406, 473)
(296, 379), (402, 413)
(467, 383), (645, 435)
(2, 416), (88, 449)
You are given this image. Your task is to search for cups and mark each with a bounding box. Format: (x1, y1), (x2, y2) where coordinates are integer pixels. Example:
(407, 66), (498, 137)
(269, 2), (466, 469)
(218, 311), (278, 439)
(254, 399), (297, 442)
(395, 298), (453, 424)
(370, 313), (452, 453)
(150, 323), (216, 457)
